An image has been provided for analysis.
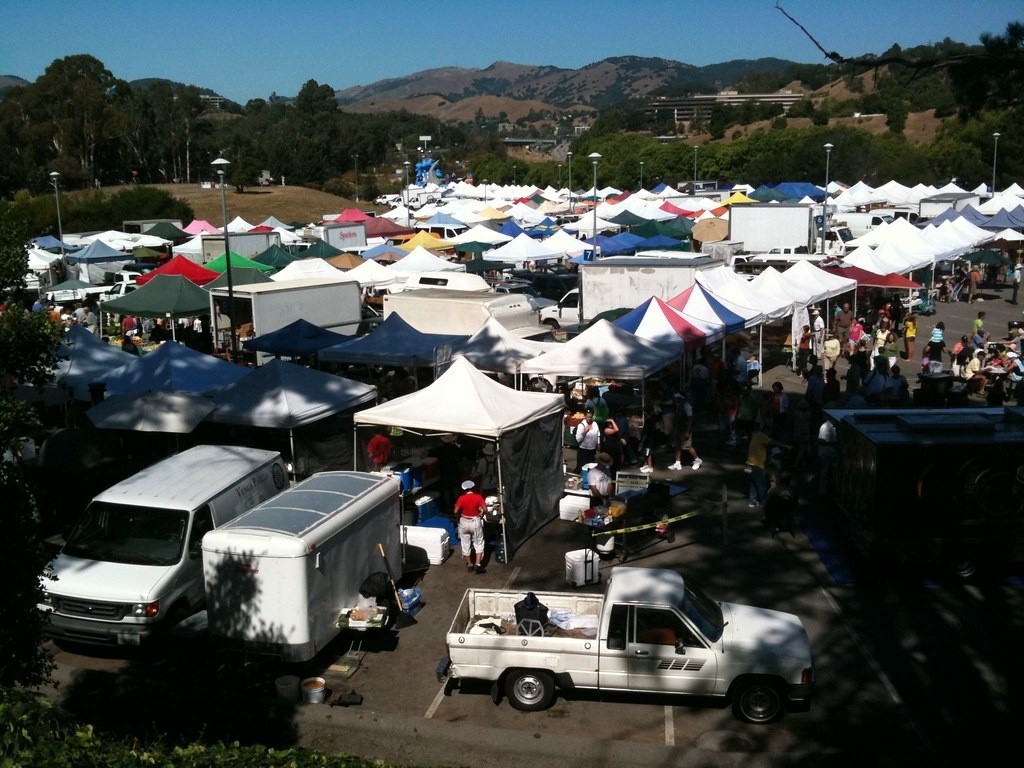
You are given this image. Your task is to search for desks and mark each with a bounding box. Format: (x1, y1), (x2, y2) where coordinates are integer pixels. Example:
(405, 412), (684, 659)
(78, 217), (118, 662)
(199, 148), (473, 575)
(574, 477), (688, 563)
(336, 605), (390, 660)
(982, 367), (1008, 389)
(399, 472), (441, 499)
(563, 488), (592, 497)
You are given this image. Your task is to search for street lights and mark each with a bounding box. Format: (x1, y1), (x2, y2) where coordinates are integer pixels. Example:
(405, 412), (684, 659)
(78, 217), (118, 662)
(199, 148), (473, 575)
(990, 131), (1002, 200)
(821, 142), (836, 254)
(564, 151), (574, 214)
(588, 150), (603, 258)
(209, 157), (238, 365)
(403, 160), (412, 230)
(694, 145), (700, 191)
(49, 170), (68, 279)
(638, 161), (645, 191)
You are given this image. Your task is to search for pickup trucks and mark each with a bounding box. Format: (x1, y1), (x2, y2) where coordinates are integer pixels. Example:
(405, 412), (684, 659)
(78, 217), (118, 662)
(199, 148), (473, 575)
(435, 567), (816, 725)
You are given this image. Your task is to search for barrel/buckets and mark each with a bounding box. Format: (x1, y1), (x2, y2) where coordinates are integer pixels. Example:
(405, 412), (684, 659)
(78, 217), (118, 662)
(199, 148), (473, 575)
(275, 674), (300, 702)
(300, 676), (326, 705)
(393, 467), (413, 491)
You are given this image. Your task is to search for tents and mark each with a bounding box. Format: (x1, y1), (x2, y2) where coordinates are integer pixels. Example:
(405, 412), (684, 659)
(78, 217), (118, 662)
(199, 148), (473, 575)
(0, 177), (1024, 372)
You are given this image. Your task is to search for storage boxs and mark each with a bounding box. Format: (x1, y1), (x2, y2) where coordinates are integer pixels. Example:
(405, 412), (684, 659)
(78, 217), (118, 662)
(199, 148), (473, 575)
(383, 446), (450, 566)
(581, 463), (610, 489)
(566, 548), (602, 589)
(559, 494), (590, 522)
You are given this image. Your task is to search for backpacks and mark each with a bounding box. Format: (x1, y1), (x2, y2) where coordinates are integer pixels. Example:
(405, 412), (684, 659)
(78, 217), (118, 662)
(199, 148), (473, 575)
(882, 316), (890, 327)
(589, 399), (608, 423)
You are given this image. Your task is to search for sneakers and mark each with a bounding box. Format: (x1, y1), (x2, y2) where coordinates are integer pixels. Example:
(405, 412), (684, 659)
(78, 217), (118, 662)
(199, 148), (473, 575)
(693, 459), (702, 470)
(668, 462), (683, 471)
(639, 465), (654, 473)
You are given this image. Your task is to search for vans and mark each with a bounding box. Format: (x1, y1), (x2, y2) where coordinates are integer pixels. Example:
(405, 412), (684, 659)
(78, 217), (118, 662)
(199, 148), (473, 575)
(35, 443), (291, 652)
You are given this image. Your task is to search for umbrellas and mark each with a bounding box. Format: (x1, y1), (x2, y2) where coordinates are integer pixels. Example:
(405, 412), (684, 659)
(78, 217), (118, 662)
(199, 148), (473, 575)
(45, 279), (99, 299)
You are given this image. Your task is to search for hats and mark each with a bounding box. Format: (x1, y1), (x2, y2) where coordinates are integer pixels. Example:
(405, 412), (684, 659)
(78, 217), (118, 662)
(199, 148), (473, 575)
(878, 309), (885, 314)
(1015, 263), (1023, 269)
(440, 434), (457, 442)
(461, 480), (475, 489)
(824, 367), (838, 375)
(674, 393), (684, 399)
(595, 453), (614, 466)
(977, 352), (986, 355)
(961, 335), (968, 341)
(1004, 343), (1017, 352)
(812, 311), (819, 315)
(482, 443), (494, 455)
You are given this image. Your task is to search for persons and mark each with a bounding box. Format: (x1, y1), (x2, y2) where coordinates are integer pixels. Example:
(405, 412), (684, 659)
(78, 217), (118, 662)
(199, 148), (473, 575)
(59, 308), (77, 333)
(385, 426), (405, 458)
(37, 276), (764, 565)
(122, 316), (136, 336)
(368, 429), (393, 472)
(82, 293), (98, 313)
(576, 407), (600, 473)
(73, 303), (85, 319)
(454, 480), (487, 572)
(82, 307), (97, 325)
(144, 319), (152, 330)
(47, 306), (59, 321)
(472, 443), (495, 495)
(599, 265), (1024, 506)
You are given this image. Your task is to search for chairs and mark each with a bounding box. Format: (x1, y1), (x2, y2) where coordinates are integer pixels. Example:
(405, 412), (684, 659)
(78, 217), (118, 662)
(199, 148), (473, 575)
(1006, 373), (1024, 403)
(959, 361), (983, 393)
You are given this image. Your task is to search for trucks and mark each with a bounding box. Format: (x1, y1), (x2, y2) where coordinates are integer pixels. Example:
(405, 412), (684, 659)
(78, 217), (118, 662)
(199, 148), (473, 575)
(727, 201), (853, 254)
(539, 248), (714, 329)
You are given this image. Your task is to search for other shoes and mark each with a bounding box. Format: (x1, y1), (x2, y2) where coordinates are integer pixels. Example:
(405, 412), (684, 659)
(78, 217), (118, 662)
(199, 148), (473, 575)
(749, 502), (760, 507)
(474, 565), (487, 574)
(468, 565), (474, 572)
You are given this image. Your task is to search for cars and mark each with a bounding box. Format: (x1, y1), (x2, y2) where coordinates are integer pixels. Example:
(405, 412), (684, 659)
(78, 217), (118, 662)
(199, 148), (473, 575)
(493, 281), (559, 313)
(751, 244), (838, 271)
(99, 280), (141, 303)
(729, 255), (760, 281)
